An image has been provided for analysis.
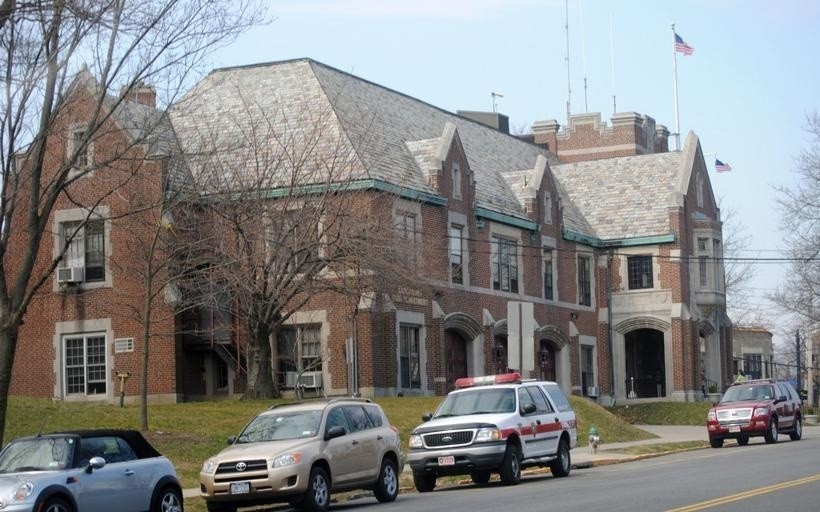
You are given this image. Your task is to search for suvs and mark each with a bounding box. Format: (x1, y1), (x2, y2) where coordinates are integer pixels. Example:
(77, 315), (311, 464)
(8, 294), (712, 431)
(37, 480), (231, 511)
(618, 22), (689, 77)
(407, 372), (578, 493)
(706, 378), (802, 448)
(199, 398), (404, 512)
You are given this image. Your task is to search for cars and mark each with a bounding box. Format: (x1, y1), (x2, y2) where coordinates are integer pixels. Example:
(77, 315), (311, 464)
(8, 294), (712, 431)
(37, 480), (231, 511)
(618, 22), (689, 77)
(0, 429), (184, 512)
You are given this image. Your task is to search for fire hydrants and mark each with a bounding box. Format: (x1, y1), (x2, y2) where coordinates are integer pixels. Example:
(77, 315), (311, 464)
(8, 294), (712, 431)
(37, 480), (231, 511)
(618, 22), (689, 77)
(587, 426), (600, 455)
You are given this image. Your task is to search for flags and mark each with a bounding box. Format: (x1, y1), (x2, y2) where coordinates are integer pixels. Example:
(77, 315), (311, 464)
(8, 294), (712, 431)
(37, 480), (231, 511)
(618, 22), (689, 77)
(674, 32), (693, 54)
(714, 159), (731, 172)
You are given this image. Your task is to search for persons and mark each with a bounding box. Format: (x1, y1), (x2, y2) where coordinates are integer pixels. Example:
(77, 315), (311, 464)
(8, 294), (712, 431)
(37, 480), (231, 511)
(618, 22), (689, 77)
(734, 368), (748, 384)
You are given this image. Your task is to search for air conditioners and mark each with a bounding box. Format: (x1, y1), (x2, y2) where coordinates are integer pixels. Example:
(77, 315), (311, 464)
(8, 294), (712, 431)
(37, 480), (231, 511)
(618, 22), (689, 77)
(57, 267), (83, 282)
